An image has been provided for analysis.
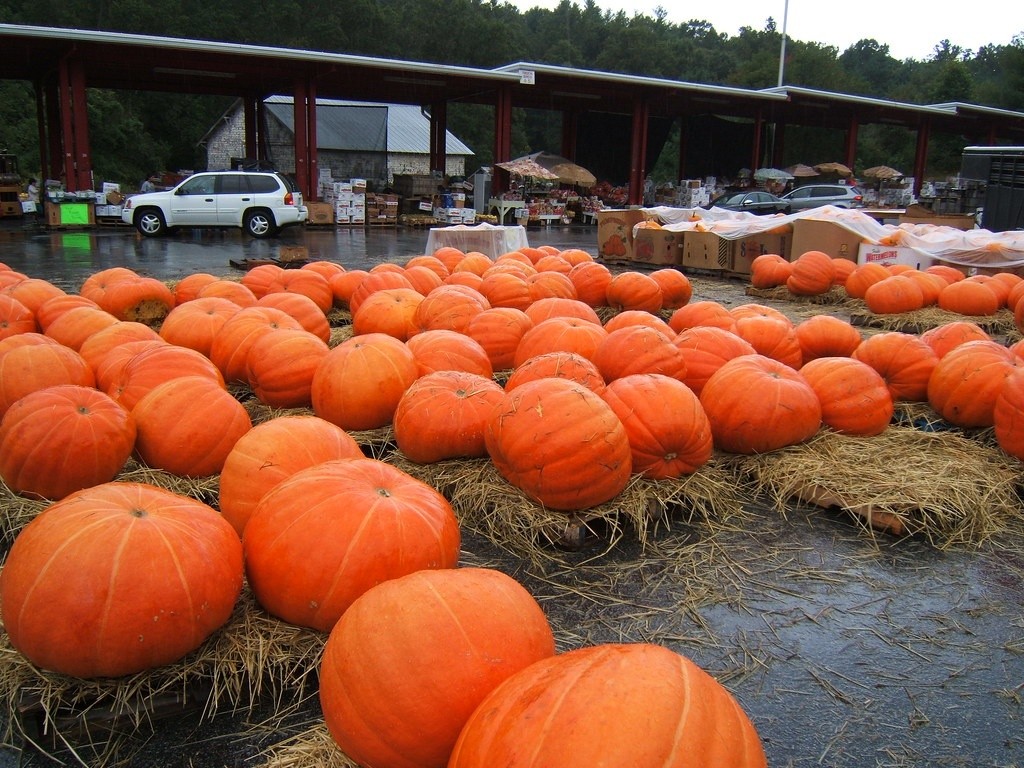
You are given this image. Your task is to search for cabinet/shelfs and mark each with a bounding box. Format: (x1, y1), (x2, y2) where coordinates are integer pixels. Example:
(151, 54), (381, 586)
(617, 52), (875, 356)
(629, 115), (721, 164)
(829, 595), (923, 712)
(525, 194), (611, 225)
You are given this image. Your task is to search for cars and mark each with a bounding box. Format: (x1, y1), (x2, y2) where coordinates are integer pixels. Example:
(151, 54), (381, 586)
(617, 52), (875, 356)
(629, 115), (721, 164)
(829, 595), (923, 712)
(700, 190), (792, 218)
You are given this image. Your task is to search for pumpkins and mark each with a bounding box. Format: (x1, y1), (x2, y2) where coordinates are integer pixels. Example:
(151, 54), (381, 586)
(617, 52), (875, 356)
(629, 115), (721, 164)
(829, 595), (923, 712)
(0, 205), (1024, 538)
(242, 458), (461, 632)
(0, 480), (244, 678)
(320, 567), (556, 768)
(448, 644), (769, 768)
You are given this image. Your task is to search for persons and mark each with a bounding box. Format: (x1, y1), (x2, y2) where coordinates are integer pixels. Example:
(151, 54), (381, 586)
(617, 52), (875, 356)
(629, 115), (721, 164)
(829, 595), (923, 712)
(140, 172), (154, 194)
(27, 177), (40, 225)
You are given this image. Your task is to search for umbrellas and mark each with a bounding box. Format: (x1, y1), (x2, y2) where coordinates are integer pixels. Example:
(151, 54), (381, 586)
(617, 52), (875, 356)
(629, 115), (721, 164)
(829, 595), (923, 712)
(515, 151), (598, 189)
(494, 157), (559, 201)
(738, 162), (903, 192)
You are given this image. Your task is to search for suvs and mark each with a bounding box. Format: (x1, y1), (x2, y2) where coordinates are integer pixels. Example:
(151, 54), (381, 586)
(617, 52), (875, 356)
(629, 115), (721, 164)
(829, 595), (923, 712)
(780, 185), (864, 210)
(121, 170), (308, 239)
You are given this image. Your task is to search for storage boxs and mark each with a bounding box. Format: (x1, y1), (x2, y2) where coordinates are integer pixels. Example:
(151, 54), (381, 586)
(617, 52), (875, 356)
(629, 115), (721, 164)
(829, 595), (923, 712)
(632, 227), (684, 264)
(645, 180), (725, 208)
(43, 180), (126, 227)
(304, 201), (334, 224)
(941, 250), (1024, 279)
(733, 222), (793, 274)
(317, 168), (399, 224)
(393, 174), (497, 224)
(790, 218), (862, 263)
(682, 232), (732, 269)
(857, 242), (952, 270)
(597, 210), (664, 260)
(899, 206), (975, 232)
(280, 246), (309, 262)
(21, 201), (37, 213)
(857, 178), (959, 206)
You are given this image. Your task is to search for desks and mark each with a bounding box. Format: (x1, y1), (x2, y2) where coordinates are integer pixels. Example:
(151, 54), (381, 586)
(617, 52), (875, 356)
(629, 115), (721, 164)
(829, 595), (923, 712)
(488, 199), (525, 225)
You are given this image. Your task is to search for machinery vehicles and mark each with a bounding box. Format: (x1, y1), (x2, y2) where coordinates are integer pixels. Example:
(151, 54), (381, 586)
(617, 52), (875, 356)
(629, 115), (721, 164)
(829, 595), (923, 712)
(0, 154), (24, 217)
